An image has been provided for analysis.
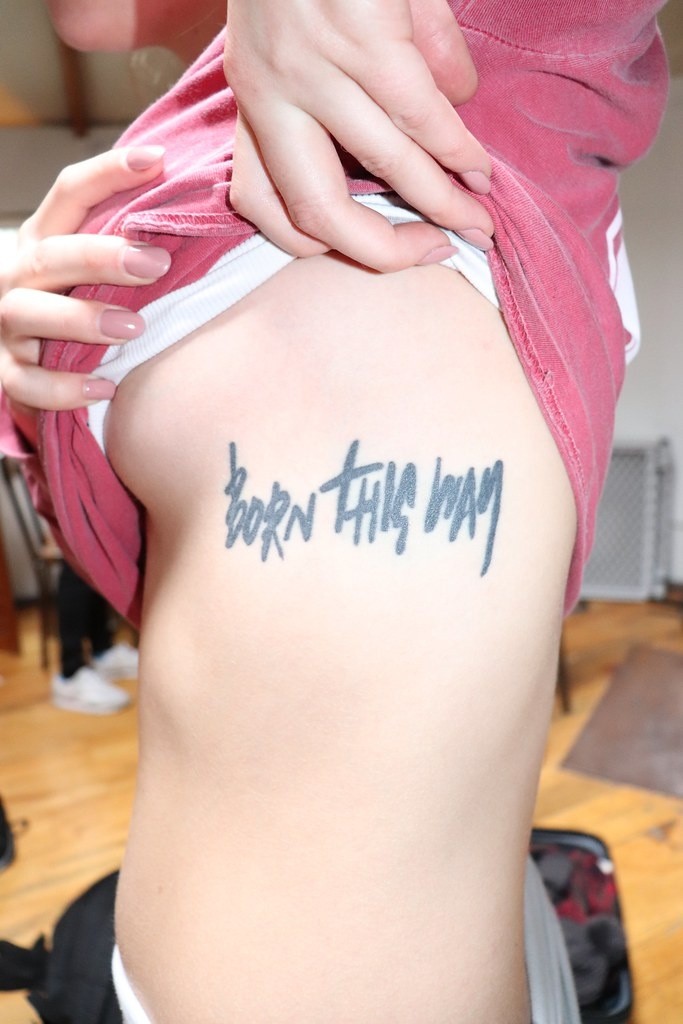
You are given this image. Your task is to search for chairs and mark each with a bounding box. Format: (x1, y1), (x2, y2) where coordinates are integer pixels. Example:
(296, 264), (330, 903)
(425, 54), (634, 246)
(0, 456), (63, 672)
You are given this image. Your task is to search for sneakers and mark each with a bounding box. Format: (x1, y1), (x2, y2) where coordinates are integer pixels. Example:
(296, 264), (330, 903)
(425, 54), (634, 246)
(95, 642), (139, 676)
(56, 665), (126, 713)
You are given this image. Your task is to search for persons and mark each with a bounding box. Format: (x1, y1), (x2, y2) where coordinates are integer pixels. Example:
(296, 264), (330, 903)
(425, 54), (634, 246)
(0, 0), (672, 1024)
